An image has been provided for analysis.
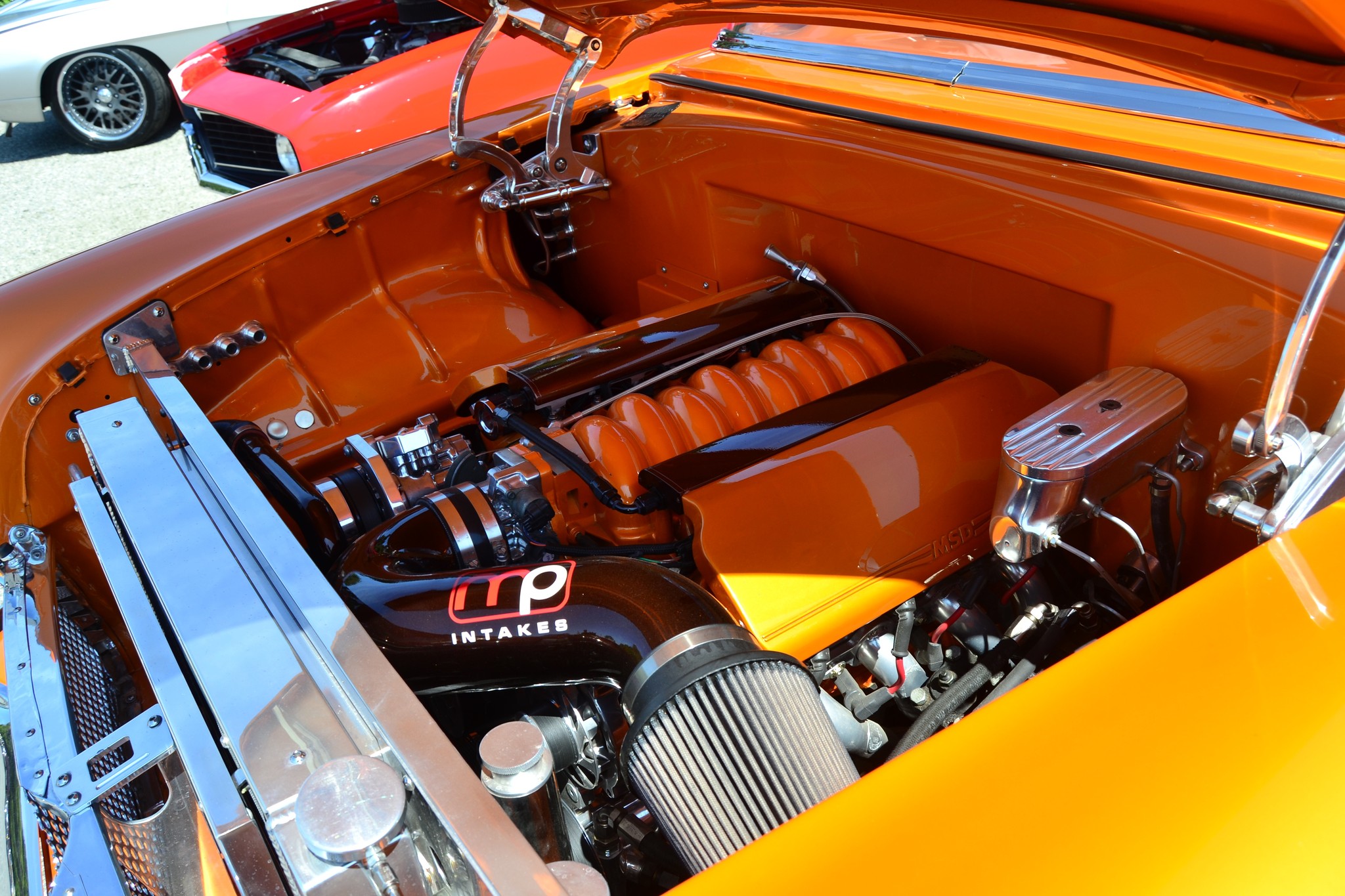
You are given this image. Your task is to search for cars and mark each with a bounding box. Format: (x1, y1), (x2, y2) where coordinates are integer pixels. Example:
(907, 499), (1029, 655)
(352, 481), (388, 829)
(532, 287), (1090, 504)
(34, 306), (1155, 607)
(0, 1), (339, 151)
(169, 1), (768, 194)
(0, 0), (1344, 892)
